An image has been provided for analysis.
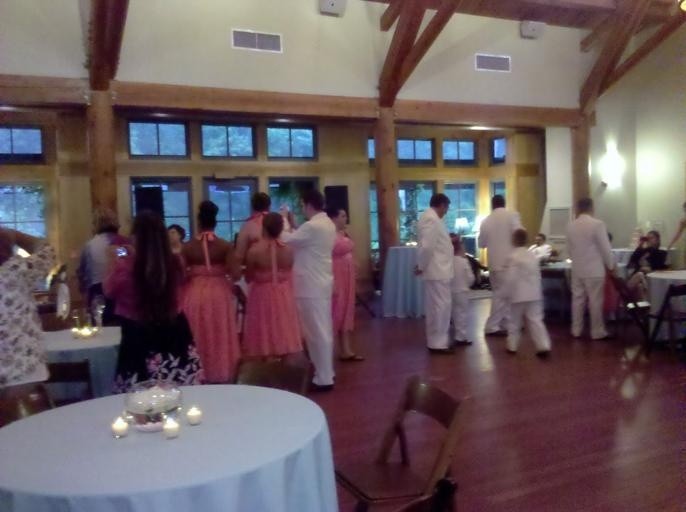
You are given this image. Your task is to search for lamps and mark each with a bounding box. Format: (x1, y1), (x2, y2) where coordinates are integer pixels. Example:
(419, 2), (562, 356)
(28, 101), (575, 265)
(453, 217), (469, 237)
(472, 216), (486, 236)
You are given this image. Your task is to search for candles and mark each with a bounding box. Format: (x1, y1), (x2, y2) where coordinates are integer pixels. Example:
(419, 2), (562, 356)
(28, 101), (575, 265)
(187, 406), (202, 425)
(111, 416), (128, 437)
(163, 417), (179, 435)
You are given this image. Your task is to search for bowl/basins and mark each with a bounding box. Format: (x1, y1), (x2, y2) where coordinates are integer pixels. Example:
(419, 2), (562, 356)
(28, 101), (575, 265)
(126, 382), (181, 431)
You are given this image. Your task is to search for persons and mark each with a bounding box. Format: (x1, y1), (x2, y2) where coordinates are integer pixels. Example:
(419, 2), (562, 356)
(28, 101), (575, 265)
(628, 229), (666, 273)
(478, 195), (525, 335)
(489, 229), (552, 357)
(667, 201), (686, 252)
(413, 193), (476, 355)
(604, 232), (648, 310)
(528, 233), (552, 259)
(568, 198), (615, 340)
(0, 226), (60, 429)
(75, 188), (366, 391)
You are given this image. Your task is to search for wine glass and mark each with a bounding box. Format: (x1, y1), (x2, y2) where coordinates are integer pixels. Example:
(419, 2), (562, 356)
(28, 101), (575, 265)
(92, 299), (105, 334)
(93, 295), (106, 309)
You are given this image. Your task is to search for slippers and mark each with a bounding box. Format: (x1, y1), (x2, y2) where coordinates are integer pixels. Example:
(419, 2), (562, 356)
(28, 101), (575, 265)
(338, 354), (364, 361)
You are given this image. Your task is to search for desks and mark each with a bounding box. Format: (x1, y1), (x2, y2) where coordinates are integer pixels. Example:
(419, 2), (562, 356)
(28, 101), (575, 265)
(540, 261), (573, 319)
(646, 270), (686, 344)
(611, 247), (676, 267)
(0, 384), (339, 512)
(382, 246), (428, 318)
(41, 326), (123, 398)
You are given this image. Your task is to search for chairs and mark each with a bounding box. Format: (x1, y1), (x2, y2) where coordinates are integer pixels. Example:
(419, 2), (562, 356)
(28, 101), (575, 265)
(0, 381), (51, 430)
(645, 284), (685, 358)
(610, 275), (651, 338)
(335, 380), (475, 512)
